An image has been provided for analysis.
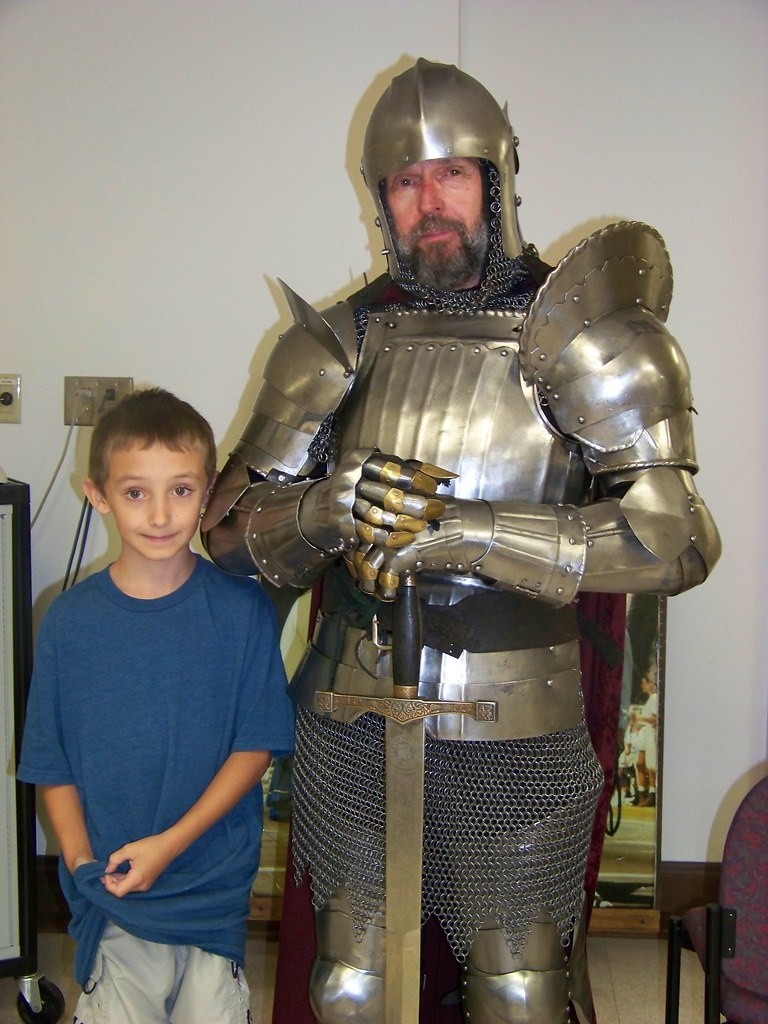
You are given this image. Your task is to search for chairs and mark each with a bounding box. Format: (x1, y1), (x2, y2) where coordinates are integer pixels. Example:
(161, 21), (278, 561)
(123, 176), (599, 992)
(666, 764), (767, 1024)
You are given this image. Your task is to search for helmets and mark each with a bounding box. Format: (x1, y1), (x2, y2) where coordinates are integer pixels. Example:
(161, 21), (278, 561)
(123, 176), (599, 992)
(360, 55), (530, 282)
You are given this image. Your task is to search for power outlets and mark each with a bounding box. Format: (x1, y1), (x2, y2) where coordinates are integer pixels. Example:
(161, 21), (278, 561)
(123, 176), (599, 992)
(63, 376), (134, 426)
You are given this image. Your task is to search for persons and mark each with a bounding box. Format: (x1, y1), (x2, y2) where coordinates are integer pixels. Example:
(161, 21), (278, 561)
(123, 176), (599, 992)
(16, 388), (296, 1024)
(201, 57), (723, 1024)
(617, 673), (658, 806)
